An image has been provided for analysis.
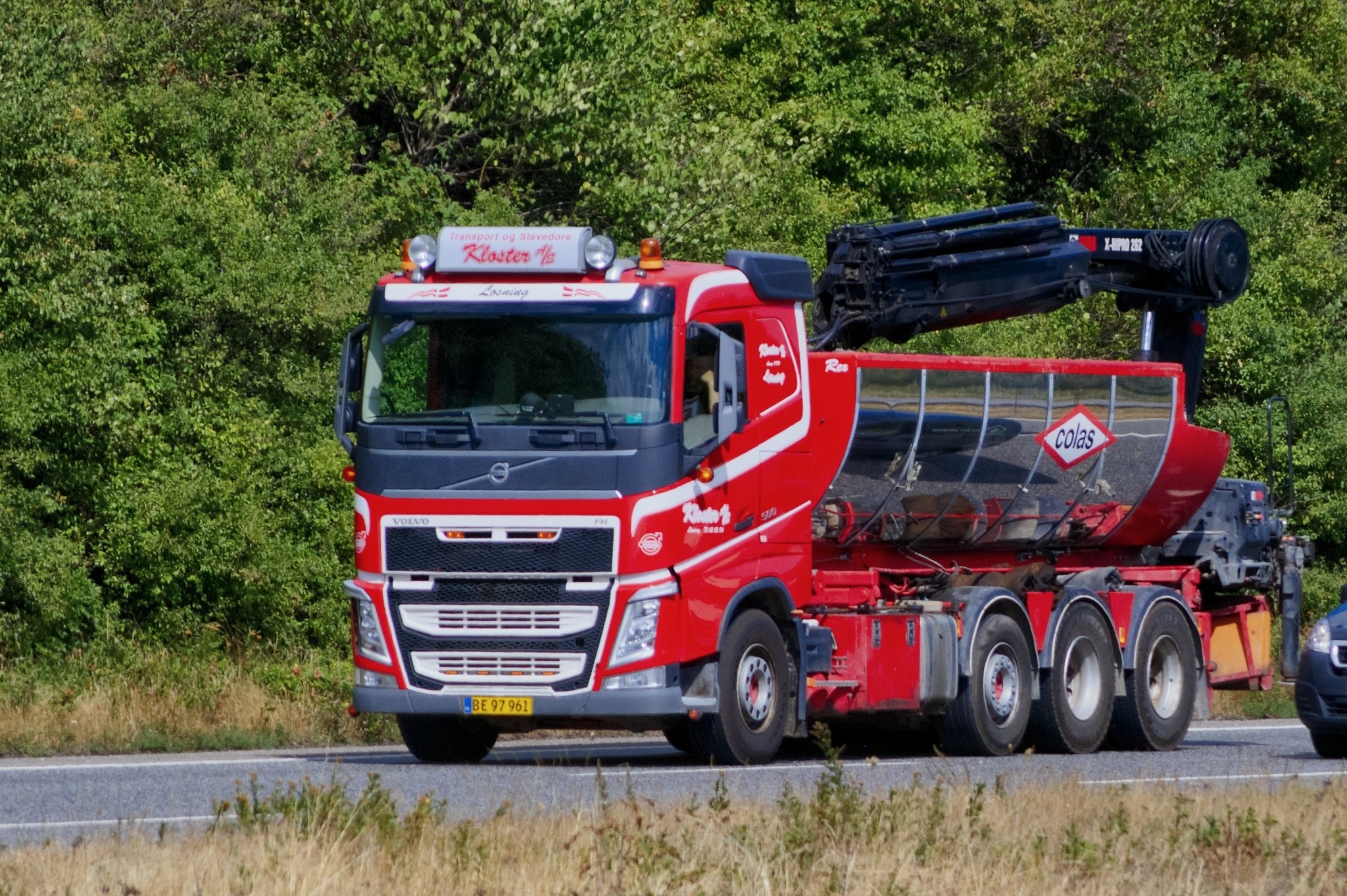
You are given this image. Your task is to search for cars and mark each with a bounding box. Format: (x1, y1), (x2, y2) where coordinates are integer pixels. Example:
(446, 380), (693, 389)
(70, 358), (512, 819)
(1295, 584), (1347, 759)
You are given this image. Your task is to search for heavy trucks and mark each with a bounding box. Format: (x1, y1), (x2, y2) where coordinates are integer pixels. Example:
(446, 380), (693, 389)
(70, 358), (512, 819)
(335, 226), (1314, 765)
